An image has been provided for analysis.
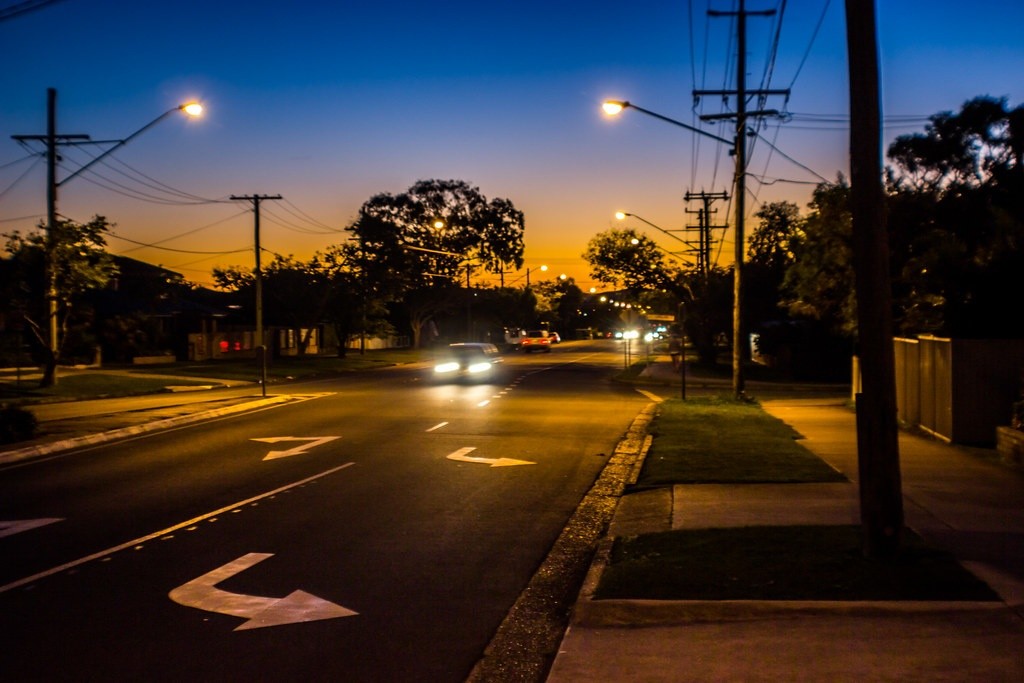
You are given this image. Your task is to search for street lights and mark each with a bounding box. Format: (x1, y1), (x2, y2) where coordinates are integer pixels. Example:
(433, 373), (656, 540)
(602, 99), (748, 398)
(44, 101), (201, 384)
(616, 211), (710, 371)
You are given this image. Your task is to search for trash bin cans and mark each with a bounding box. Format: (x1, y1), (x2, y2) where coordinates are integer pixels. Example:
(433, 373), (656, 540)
(853, 336), (998, 449)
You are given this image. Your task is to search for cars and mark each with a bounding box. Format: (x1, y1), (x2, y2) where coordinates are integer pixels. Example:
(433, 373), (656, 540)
(522, 330), (550, 352)
(437, 343), (502, 380)
(588, 325), (673, 342)
(549, 332), (561, 343)
